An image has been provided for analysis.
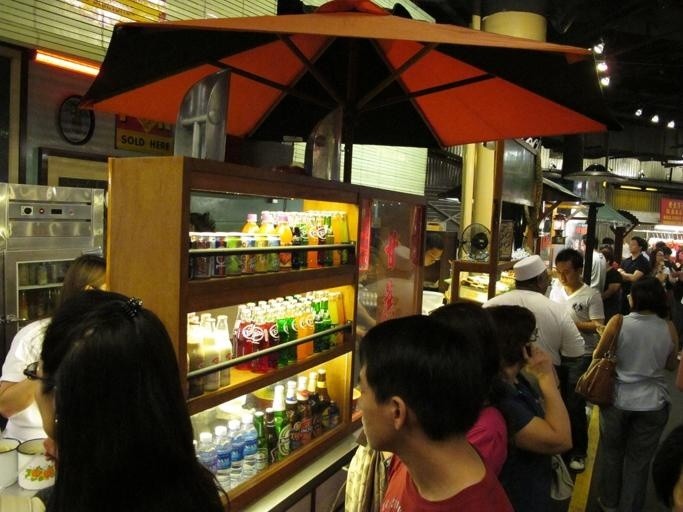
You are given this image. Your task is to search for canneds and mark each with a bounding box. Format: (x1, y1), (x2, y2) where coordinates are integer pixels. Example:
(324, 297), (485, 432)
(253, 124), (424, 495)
(189, 231), (280, 279)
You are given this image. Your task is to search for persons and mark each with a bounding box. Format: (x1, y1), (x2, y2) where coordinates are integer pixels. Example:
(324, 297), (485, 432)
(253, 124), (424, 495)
(652, 353), (683, 512)
(381, 302), (509, 478)
(0, 254), (106, 444)
(676, 251), (683, 352)
(25, 291), (231, 512)
(358, 314), (515, 512)
(549, 248), (605, 473)
(662, 248), (677, 297)
(617, 236), (650, 316)
(424, 233), (446, 267)
(591, 275), (678, 511)
(580, 235), (606, 299)
(647, 250), (678, 322)
(599, 247), (622, 326)
(483, 306), (572, 512)
(602, 237), (614, 257)
(481, 255), (585, 390)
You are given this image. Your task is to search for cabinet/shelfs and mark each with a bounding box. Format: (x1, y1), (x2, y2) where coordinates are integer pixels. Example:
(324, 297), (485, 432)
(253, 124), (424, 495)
(105, 155), (429, 512)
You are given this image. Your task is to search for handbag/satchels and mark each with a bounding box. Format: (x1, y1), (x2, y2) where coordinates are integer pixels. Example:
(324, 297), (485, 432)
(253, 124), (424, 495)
(513, 370), (575, 501)
(575, 314), (625, 406)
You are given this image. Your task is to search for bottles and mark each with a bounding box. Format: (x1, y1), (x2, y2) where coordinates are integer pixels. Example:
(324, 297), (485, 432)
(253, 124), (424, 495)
(243, 210), (351, 274)
(233, 290), (347, 373)
(194, 413), (263, 494)
(263, 368), (342, 464)
(187, 311), (232, 396)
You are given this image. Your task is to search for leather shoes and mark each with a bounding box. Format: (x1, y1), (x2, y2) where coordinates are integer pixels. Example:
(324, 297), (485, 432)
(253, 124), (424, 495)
(568, 454), (587, 473)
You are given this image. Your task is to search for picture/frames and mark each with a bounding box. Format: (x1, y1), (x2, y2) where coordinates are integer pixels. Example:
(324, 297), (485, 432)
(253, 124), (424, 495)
(0, 40), (32, 184)
(37, 145), (111, 191)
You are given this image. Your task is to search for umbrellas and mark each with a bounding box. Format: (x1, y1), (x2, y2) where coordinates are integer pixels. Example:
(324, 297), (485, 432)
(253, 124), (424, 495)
(79, 0), (615, 186)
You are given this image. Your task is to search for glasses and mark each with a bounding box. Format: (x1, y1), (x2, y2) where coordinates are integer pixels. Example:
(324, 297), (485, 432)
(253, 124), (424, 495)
(627, 294), (632, 298)
(22, 360), (50, 384)
(655, 254), (665, 257)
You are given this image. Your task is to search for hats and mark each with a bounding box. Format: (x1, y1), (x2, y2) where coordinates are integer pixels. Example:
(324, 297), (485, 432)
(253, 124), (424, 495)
(513, 255), (546, 282)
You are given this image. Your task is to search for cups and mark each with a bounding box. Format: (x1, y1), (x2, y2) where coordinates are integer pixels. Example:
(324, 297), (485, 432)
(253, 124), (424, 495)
(0, 438), (20, 489)
(16, 438), (55, 491)
